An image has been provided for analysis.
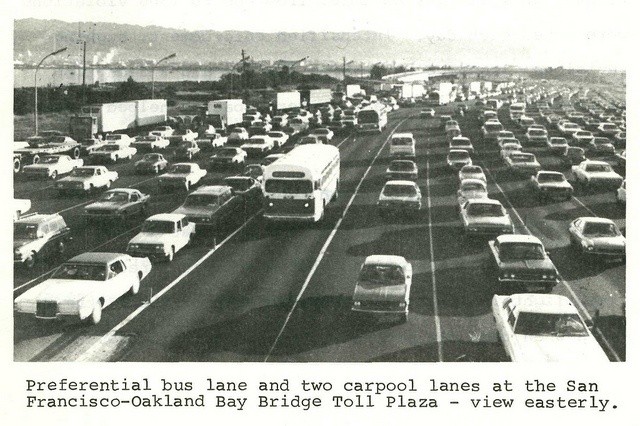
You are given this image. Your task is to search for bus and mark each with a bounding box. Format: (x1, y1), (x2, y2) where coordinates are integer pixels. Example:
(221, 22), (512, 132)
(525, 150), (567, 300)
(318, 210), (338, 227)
(430, 90), (448, 106)
(263, 143), (340, 227)
(509, 103), (525, 121)
(358, 102), (388, 133)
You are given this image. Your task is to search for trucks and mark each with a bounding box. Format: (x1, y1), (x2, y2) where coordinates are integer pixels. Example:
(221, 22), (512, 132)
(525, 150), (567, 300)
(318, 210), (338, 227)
(203, 99), (243, 136)
(14, 130), (81, 174)
(69, 99), (167, 141)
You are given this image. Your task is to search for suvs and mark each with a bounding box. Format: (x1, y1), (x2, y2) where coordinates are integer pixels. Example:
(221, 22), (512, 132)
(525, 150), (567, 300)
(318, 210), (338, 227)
(80, 139), (106, 155)
(500, 144), (521, 160)
(506, 153), (542, 176)
(499, 138), (522, 152)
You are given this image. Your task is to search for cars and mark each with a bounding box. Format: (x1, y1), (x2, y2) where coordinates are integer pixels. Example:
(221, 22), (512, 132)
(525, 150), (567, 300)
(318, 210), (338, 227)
(550, 137), (567, 155)
(128, 213), (196, 262)
(458, 179), (488, 204)
(243, 98), (359, 135)
(196, 133), (227, 148)
(441, 116), (452, 127)
(23, 154), (83, 180)
(475, 82), (625, 139)
(446, 120), (459, 133)
(532, 171), (573, 201)
(149, 126), (174, 139)
(350, 255), (412, 323)
(390, 133), (415, 158)
(493, 294), (610, 362)
(171, 130), (198, 143)
(572, 161), (623, 187)
(379, 181), (421, 218)
(447, 128), (461, 144)
(209, 147), (248, 170)
(568, 148), (585, 164)
(172, 184), (239, 234)
(244, 163), (268, 184)
(313, 128), (334, 143)
(84, 188), (151, 224)
(268, 131), (289, 147)
(295, 135), (322, 148)
(57, 166), (118, 197)
(589, 137), (615, 155)
(230, 128), (248, 140)
(450, 137), (474, 159)
(241, 135), (274, 156)
(420, 108), (435, 118)
(14, 252), (151, 326)
(224, 176), (261, 203)
(14, 196), (31, 220)
(13, 213), (71, 268)
(130, 136), (170, 150)
(88, 145), (137, 163)
(461, 199), (514, 235)
(174, 141), (200, 161)
(261, 154), (285, 171)
(489, 234), (560, 291)
(134, 153), (167, 174)
(446, 150), (472, 171)
(105, 133), (130, 146)
(156, 163), (207, 191)
(460, 166), (486, 181)
(569, 217), (626, 261)
(386, 160), (417, 182)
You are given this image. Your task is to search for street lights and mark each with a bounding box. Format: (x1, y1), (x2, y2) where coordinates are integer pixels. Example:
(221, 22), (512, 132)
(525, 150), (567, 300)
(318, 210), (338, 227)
(289, 56), (310, 71)
(35, 46), (68, 138)
(229, 56), (250, 100)
(152, 53), (176, 100)
